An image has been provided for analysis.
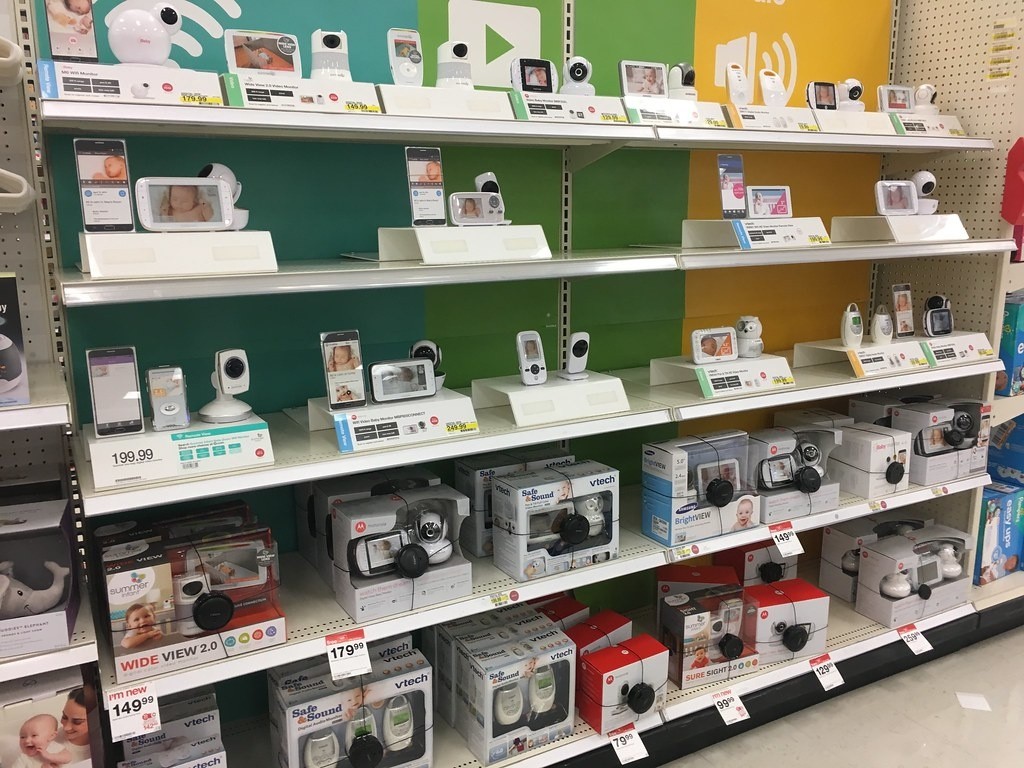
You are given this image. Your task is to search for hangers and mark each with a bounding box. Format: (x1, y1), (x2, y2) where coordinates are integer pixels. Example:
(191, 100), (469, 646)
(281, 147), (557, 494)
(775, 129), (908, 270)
(0, 36), (24, 87)
(0, 168), (35, 213)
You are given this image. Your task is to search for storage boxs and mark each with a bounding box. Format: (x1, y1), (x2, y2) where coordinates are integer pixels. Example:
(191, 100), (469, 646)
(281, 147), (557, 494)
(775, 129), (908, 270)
(974, 414), (1024, 587)
(81, 445), (670, 768)
(644, 394), (992, 693)
(0, 498), (80, 657)
(997, 287), (1024, 398)
(0, 464), (64, 514)
(0, 667), (93, 768)
(0, 271), (31, 407)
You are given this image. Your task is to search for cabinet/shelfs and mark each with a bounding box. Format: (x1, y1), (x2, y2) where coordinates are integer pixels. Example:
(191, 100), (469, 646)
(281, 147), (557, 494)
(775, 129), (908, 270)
(565, 1), (1017, 767)
(0, 1), (107, 768)
(31, 1), (565, 768)
(976, 262), (1024, 639)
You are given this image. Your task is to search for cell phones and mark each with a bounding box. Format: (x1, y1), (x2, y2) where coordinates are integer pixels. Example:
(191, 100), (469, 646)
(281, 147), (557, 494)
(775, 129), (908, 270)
(369, 358), (436, 404)
(74, 138), (136, 233)
(224, 29), (302, 79)
(807, 82), (839, 111)
(145, 364), (190, 432)
(186, 423), (956, 591)
(926, 308), (954, 337)
(135, 176), (235, 232)
(717, 154), (746, 220)
(86, 346), (145, 439)
(45, 0), (99, 63)
(692, 327), (738, 364)
(746, 185), (793, 220)
(875, 180), (918, 216)
(878, 86), (915, 114)
(510, 58), (559, 95)
(319, 330), (367, 413)
(404, 146), (447, 228)
(619, 60), (668, 98)
(891, 284), (914, 337)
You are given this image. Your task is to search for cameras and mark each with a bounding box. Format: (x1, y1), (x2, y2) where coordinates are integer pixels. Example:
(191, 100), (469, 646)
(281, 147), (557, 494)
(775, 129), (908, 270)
(527, 500), (575, 544)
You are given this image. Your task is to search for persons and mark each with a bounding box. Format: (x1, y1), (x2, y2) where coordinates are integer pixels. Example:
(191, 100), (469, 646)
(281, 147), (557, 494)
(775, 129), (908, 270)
(65, 0), (93, 34)
(418, 162), (440, 182)
(817, 86), (834, 104)
(92, 157), (125, 180)
(525, 341), (537, 354)
(930, 428), (946, 446)
(701, 337), (717, 357)
(12, 687), (92, 768)
(328, 345), (362, 401)
(980, 554), (1020, 586)
(460, 199), (481, 217)
(690, 647), (709, 670)
(887, 187), (908, 209)
(722, 174), (733, 189)
(638, 69), (662, 93)
(382, 368), (413, 393)
(161, 186), (213, 223)
(754, 192), (770, 214)
(889, 91), (907, 102)
(121, 603), (162, 648)
(896, 294), (911, 331)
(530, 67), (547, 85)
(729, 499), (755, 532)
(553, 480), (572, 503)
